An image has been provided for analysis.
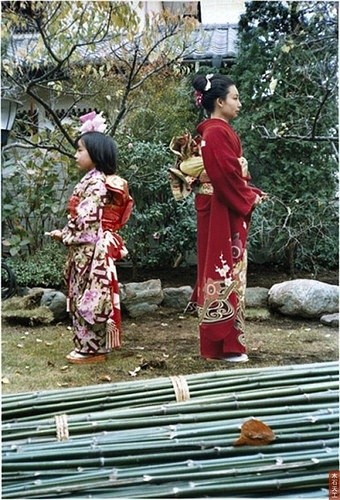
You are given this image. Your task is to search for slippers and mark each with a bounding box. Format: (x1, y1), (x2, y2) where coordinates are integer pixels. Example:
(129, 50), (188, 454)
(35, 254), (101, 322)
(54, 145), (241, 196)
(206, 353), (249, 364)
(64, 351), (106, 364)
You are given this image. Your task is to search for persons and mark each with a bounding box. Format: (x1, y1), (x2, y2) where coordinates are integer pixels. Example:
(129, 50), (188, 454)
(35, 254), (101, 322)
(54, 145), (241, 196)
(168, 74), (270, 363)
(51, 132), (134, 363)
